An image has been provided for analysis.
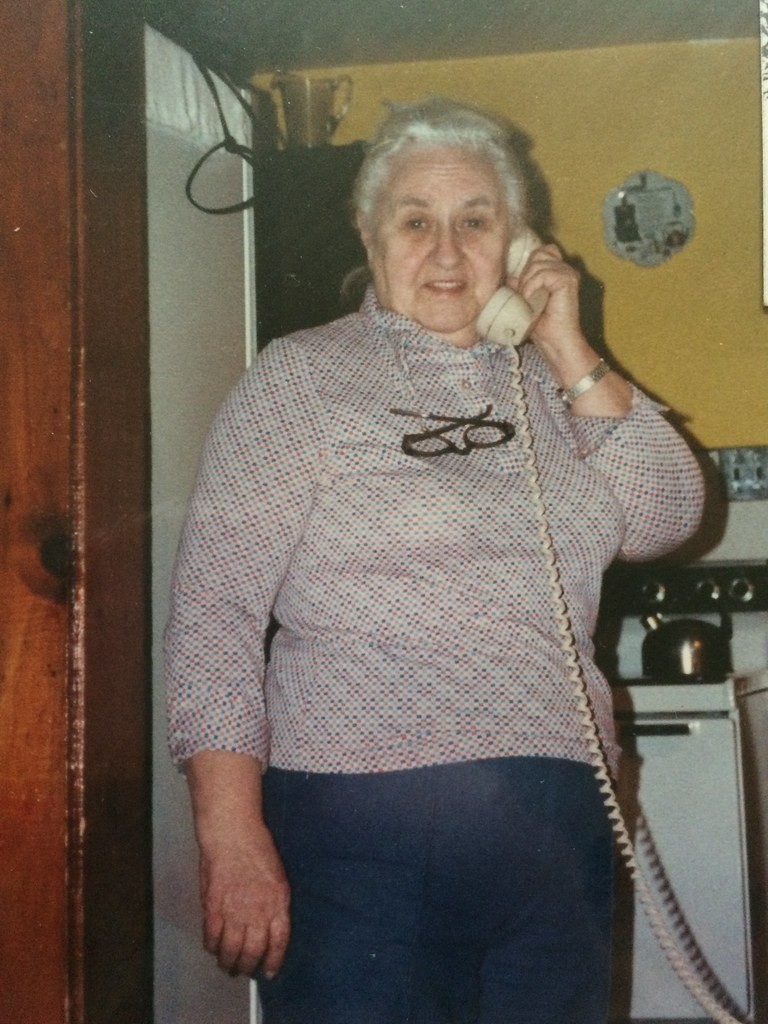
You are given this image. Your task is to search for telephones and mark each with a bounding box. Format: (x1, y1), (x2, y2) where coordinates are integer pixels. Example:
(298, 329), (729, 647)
(475, 222), (551, 348)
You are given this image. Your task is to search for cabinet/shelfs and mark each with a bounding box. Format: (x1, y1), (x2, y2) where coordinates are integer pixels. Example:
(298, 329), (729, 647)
(605, 668), (768, 1024)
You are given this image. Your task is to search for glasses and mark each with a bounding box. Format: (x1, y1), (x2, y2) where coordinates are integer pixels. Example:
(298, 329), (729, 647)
(390, 403), (517, 457)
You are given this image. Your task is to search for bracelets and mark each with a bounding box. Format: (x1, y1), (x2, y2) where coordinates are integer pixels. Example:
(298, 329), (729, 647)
(562, 360), (611, 406)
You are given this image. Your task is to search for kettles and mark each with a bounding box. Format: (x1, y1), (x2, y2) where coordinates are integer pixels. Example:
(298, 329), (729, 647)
(639, 599), (732, 684)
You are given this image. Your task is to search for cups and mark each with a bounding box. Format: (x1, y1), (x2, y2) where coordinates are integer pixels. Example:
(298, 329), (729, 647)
(280, 74), (353, 147)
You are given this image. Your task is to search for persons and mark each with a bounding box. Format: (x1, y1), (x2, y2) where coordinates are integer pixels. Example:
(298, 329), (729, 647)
(162, 95), (705, 1023)
